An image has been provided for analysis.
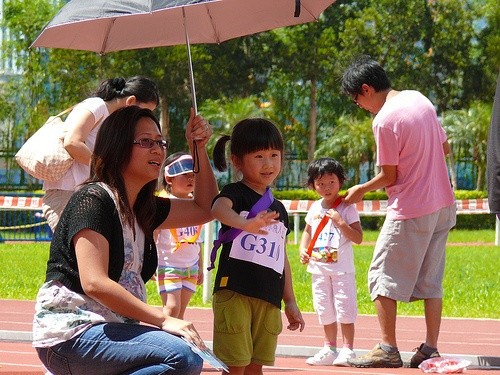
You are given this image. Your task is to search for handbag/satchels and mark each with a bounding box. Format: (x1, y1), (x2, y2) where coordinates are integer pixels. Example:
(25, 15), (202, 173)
(15, 104), (104, 181)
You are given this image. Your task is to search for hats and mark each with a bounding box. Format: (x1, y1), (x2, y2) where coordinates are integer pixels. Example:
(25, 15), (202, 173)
(166, 155), (194, 177)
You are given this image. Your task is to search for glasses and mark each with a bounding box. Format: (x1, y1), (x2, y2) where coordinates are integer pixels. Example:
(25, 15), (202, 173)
(352, 93), (358, 104)
(133, 140), (167, 149)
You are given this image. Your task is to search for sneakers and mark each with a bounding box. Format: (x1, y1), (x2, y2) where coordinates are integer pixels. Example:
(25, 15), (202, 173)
(333, 348), (356, 367)
(403, 344), (441, 368)
(349, 344), (403, 368)
(307, 346), (337, 366)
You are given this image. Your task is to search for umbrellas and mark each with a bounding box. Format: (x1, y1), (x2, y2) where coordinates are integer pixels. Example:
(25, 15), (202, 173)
(29, 0), (336, 141)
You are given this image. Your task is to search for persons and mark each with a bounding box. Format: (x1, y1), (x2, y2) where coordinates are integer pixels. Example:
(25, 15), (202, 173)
(150, 152), (204, 319)
(341, 55), (456, 368)
(485, 76), (500, 217)
(42, 76), (159, 235)
(298, 158), (362, 366)
(211, 118), (305, 375)
(32, 105), (220, 375)
